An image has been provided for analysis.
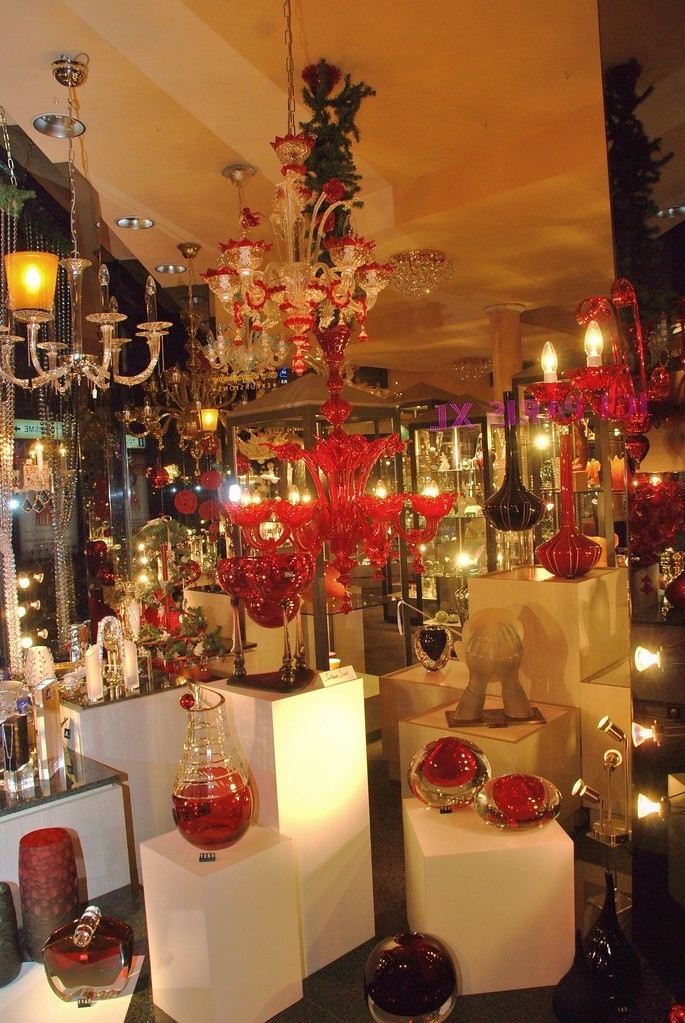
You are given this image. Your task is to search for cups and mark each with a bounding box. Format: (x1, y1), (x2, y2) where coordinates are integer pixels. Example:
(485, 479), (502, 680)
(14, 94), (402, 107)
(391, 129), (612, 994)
(0, 881), (22, 989)
(18, 828), (80, 963)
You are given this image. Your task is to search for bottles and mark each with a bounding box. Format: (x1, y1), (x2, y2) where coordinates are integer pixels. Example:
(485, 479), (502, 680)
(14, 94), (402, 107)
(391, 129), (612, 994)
(482, 391), (545, 532)
(534, 434), (602, 577)
(171, 680), (255, 851)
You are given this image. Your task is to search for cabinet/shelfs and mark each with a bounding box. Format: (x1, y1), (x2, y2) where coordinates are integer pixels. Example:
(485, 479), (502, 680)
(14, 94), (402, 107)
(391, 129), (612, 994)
(528, 410), (627, 571)
(379, 415), (527, 628)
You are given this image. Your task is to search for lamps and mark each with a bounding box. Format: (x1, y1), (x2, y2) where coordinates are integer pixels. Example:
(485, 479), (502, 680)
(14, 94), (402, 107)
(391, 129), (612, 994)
(219, 54), (460, 615)
(200, 0), (397, 380)
(0, 59), (175, 400)
(10, 412), (69, 514)
(117, 243), (257, 484)
(630, 644), (685, 820)
(16, 570), (49, 649)
(524, 277), (672, 467)
(628, 472), (685, 559)
(201, 161), (291, 383)
(570, 715), (633, 848)
